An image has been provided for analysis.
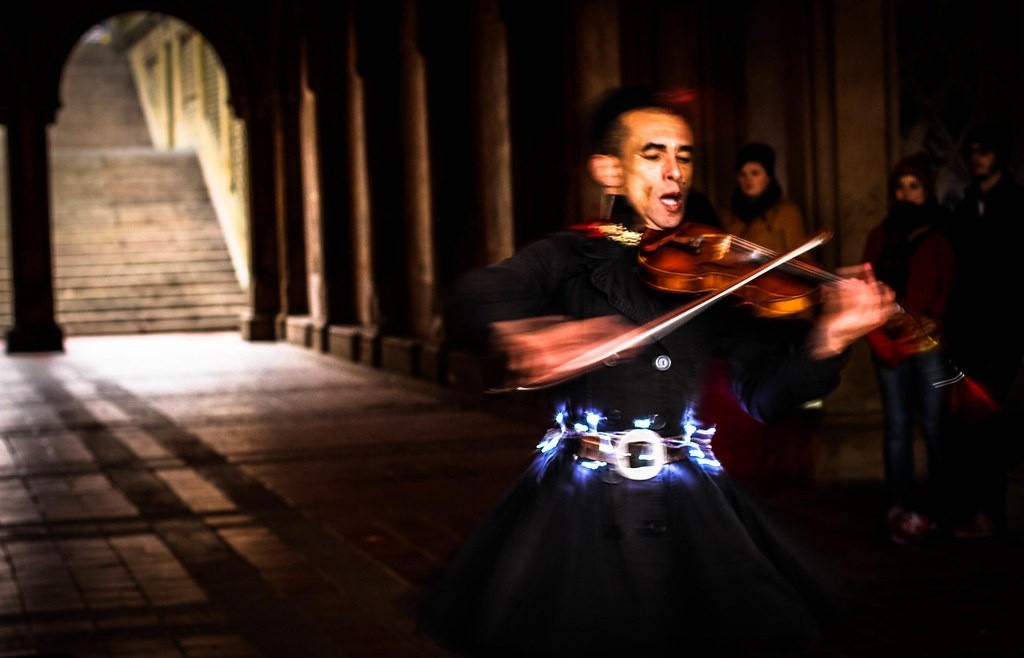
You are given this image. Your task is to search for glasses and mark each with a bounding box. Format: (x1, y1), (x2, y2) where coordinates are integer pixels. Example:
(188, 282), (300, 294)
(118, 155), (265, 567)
(965, 142), (989, 156)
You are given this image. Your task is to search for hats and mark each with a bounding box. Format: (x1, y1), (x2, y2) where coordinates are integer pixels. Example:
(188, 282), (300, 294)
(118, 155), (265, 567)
(891, 151), (936, 197)
(734, 141), (776, 180)
(966, 117), (1012, 166)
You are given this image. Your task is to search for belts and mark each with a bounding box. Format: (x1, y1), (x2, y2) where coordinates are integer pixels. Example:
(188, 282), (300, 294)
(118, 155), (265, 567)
(565, 431), (689, 481)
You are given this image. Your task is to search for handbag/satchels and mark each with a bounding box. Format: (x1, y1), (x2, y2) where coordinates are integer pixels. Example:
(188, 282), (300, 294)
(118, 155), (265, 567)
(948, 364), (1000, 421)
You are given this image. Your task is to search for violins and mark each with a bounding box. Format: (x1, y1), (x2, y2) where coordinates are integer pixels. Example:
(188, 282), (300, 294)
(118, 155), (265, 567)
(635, 217), (940, 354)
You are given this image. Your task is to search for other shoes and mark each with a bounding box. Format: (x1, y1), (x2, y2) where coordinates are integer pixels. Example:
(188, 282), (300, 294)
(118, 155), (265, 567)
(957, 510), (996, 543)
(885, 506), (937, 543)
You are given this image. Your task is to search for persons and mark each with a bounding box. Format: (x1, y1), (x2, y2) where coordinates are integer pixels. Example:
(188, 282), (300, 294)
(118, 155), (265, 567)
(686, 124), (1024, 559)
(436, 86), (897, 658)
